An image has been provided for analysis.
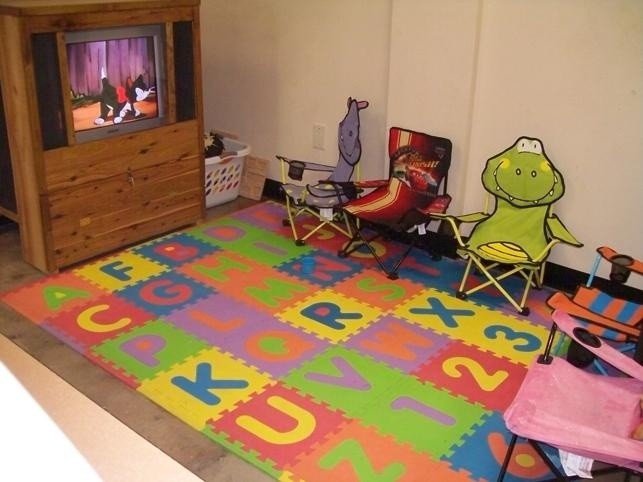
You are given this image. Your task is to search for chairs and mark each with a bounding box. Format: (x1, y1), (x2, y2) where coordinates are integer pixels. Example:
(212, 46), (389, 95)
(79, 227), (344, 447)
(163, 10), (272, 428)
(428, 136), (584, 317)
(547, 245), (641, 380)
(498, 308), (642, 481)
(336, 127), (455, 280)
(276, 97), (368, 246)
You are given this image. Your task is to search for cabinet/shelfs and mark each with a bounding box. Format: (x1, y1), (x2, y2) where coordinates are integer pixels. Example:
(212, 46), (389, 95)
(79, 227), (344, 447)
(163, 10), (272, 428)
(2, 2), (209, 280)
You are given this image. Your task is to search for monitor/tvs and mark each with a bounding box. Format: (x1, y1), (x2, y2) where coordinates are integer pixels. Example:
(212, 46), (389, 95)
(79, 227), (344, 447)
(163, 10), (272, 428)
(62, 24), (168, 144)
